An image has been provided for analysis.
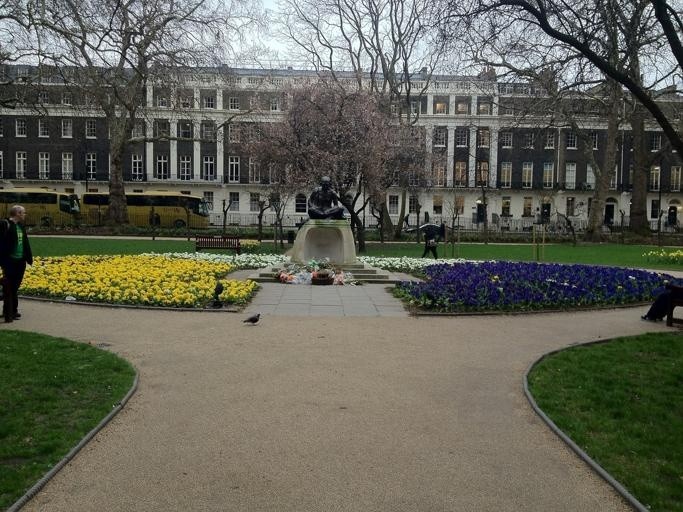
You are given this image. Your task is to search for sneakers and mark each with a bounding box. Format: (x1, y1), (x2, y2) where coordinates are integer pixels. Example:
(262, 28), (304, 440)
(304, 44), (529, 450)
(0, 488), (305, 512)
(641, 314), (663, 321)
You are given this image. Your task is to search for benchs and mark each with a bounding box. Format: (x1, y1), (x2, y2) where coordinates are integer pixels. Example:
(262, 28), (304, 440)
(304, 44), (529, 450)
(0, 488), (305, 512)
(666, 285), (683, 326)
(196, 234), (241, 255)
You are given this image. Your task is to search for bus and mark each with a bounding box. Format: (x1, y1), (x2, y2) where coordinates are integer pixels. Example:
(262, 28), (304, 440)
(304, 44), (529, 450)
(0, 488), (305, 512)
(0, 188), (80, 233)
(79, 192), (213, 229)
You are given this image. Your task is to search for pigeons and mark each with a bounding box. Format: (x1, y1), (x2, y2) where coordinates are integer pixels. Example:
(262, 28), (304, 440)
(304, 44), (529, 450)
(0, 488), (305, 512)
(243, 313), (261, 326)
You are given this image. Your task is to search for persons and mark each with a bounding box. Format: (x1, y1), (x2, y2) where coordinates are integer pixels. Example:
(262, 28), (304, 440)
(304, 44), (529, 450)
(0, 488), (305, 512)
(534, 207), (541, 224)
(0, 205), (33, 322)
(307, 176), (345, 220)
(639, 282), (683, 323)
(421, 227), (439, 259)
(148, 213), (161, 234)
(436, 220), (447, 242)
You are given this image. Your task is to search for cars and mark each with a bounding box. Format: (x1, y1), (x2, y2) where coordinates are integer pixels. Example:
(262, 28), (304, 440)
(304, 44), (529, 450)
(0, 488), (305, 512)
(405, 223), (455, 242)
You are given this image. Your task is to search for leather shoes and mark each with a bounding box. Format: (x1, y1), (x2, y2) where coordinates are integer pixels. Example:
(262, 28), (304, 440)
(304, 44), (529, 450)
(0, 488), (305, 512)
(6, 313), (20, 322)
